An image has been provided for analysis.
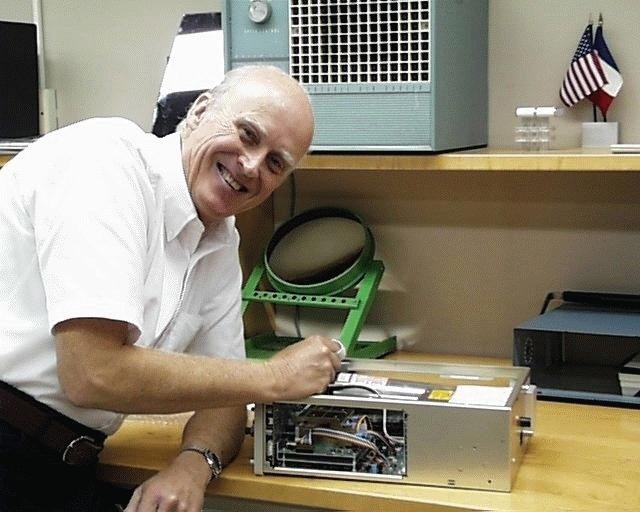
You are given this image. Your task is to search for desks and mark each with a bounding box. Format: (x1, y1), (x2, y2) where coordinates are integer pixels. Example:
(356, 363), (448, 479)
(0, 133), (639, 512)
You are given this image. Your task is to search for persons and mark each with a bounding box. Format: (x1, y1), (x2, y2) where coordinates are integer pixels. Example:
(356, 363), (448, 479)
(0, 64), (347, 512)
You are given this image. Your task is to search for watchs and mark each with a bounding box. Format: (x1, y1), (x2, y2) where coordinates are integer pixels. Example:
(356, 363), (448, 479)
(175, 442), (224, 482)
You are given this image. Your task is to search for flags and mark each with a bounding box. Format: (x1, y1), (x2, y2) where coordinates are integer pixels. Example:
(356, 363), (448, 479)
(556, 25), (607, 109)
(592, 26), (624, 119)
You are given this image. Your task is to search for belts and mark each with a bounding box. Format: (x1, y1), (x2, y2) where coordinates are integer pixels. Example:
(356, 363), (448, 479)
(0, 388), (105, 470)
